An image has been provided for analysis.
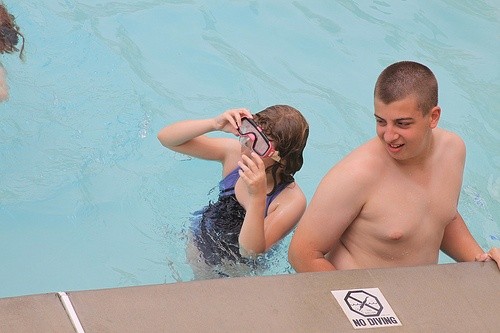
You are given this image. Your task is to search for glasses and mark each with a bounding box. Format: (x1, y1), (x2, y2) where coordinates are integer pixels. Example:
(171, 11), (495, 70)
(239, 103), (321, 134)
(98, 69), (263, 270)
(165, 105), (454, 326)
(235, 117), (283, 160)
